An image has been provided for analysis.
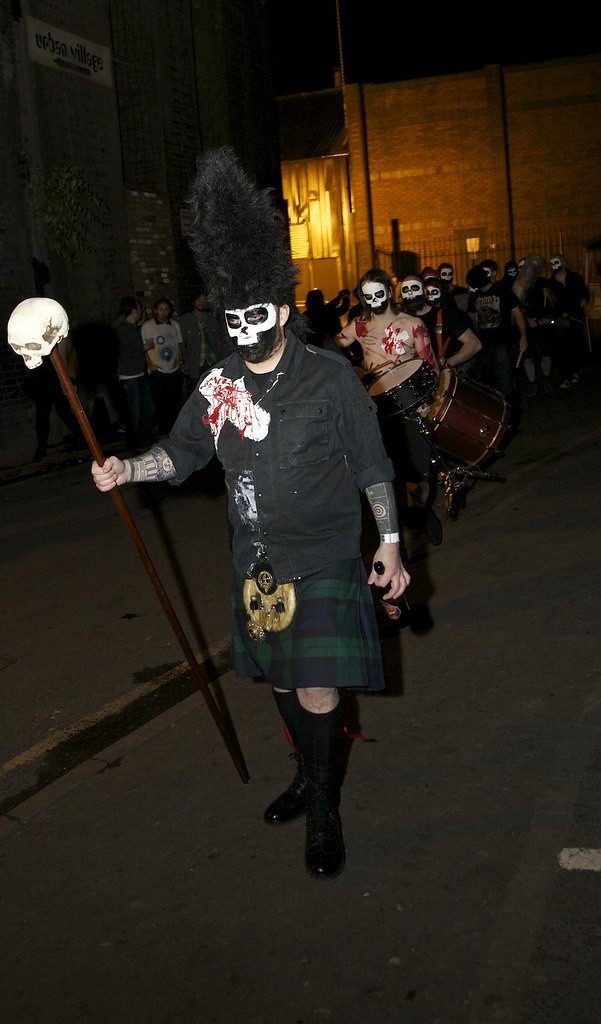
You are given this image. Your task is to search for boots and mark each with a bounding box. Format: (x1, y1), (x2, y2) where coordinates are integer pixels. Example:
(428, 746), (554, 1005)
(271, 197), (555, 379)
(263, 749), (312, 825)
(305, 776), (347, 881)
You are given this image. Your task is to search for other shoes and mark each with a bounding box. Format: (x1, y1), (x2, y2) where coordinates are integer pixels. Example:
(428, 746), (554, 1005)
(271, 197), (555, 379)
(400, 548), (408, 565)
(560, 379), (572, 388)
(572, 372), (578, 382)
(542, 380), (555, 396)
(418, 506), (442, 546)
(527, 382), (537, 398)
(32, 448), (47, 461)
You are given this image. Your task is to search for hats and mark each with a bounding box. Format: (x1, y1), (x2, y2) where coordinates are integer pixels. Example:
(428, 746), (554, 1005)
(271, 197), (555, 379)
(182, 147), (313, 341)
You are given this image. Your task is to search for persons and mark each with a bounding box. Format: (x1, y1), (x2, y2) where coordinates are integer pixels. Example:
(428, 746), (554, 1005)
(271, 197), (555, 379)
(90, 274), (410, 882)
(300, 255), (591, 566)
(112, 291), (224, 460)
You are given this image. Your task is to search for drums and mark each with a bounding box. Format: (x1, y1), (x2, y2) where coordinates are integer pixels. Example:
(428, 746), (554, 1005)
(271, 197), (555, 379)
(425, 367), (513, 469)
(367, 356), (438, 420)
